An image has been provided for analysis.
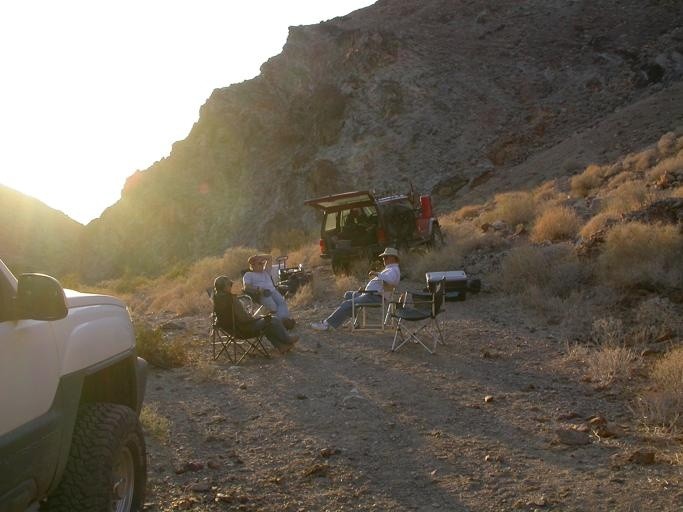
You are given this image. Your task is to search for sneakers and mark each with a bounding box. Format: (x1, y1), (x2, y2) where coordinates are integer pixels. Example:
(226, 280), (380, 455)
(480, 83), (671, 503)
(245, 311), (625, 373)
(276, 335), (299, 353)
(343, 318), (360, 329)
(310, 320), (329, 331)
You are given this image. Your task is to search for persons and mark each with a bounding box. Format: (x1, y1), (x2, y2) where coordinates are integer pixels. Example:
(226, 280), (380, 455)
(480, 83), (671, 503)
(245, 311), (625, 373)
(213, 275), (299, 354)
(309, 247), (401, 331)
(242, 255), (296, 329)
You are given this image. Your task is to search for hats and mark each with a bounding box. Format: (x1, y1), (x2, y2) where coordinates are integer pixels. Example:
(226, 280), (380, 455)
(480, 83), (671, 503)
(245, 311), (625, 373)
(378, 248), (398, 257)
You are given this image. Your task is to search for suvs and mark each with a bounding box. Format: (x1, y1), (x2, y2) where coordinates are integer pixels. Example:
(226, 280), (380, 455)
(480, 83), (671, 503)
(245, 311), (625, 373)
(302, 189), (445, 281)
(1, 256), (151, 512)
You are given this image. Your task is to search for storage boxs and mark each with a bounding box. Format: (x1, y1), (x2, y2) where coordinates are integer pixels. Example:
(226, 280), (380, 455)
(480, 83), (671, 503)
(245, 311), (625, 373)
(425, 270), (466, 301)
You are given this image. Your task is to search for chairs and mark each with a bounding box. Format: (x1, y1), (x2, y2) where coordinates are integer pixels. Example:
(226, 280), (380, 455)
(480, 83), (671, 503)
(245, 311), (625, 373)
(212, 294), (269, 365)
(241, 269), (266, 317)
(351, 280), (394, 331)
(390, 277), (446, 354)
